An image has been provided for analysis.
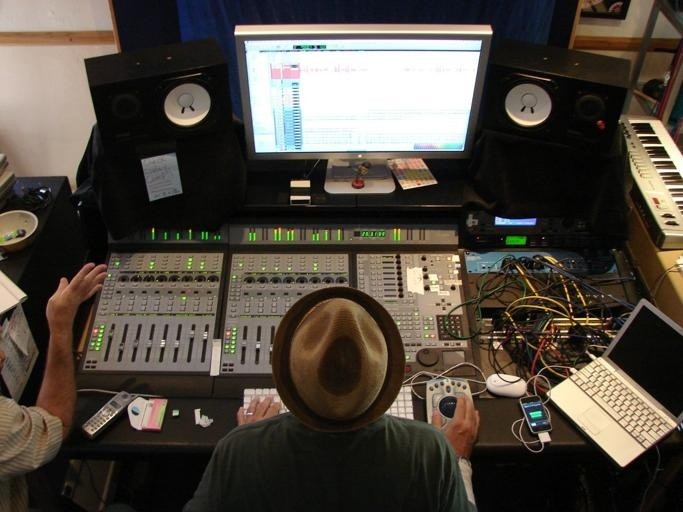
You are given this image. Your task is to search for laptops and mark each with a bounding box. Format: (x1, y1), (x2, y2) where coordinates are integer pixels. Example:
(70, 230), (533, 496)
(545, 299), (683, 469)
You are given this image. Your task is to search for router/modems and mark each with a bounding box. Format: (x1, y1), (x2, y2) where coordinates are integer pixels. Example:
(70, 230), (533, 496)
(289, 180), (311, 205)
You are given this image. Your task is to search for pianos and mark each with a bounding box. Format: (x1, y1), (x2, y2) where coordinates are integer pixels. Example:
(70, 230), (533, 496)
(619, 115), (683, 249)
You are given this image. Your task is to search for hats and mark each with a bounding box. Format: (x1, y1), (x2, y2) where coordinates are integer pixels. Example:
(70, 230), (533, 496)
(272, 287), (405, 433)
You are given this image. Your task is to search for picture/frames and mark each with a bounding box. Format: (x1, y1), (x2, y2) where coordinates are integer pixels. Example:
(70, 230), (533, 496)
(580, 0), (631, 21)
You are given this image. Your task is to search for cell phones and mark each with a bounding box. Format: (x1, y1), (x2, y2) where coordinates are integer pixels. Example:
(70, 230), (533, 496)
(81, 390), (139, 439)
(519, 396), (553, 434)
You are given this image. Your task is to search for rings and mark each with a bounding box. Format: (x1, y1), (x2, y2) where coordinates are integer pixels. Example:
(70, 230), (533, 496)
(246, 412), (253, 416)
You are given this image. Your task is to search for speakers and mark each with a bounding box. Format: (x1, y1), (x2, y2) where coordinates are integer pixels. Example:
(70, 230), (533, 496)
(492, 41), (633, 150)
(85, 40), (232, 143)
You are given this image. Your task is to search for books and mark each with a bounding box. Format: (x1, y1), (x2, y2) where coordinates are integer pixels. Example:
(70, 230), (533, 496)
(0, 271), (28, 315)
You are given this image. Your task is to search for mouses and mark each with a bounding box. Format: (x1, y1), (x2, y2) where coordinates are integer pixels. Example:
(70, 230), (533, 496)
(425, 379), (474, 427)
(486, 374), (527, 397)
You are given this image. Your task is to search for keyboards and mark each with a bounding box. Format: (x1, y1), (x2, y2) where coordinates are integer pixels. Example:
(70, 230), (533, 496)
(243, 386), (415, 422)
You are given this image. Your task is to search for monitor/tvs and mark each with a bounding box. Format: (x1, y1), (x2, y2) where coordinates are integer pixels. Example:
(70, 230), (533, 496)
(234, 24), (493, 196)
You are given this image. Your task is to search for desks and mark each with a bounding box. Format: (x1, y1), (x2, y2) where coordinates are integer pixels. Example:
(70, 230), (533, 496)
(0, 176), (73, 286)
(11, 216), (650, 460)
(622, 1), (682, 148)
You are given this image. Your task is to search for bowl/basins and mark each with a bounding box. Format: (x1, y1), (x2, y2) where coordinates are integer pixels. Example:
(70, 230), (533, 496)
(0, 209), (39, 253)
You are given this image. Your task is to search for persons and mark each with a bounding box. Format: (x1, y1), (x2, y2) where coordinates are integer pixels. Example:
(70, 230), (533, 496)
(184, 285), (481, 511)
(0, 261), (110, 512)
